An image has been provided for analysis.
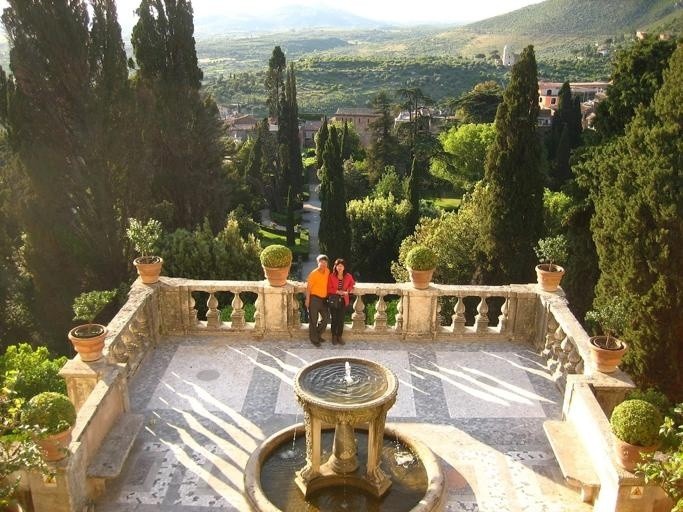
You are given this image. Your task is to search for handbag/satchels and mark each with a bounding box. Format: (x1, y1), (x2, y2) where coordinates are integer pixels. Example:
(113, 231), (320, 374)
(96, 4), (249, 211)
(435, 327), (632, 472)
(328, 295), (343, 312)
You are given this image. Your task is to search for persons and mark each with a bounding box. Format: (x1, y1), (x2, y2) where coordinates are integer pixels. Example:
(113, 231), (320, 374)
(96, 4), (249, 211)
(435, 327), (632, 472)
(304, 254), (331, 348)
(327, 258), (354, 346)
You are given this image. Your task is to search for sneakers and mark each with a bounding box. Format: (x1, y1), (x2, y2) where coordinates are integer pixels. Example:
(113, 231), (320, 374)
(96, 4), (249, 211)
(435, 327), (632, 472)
(337, 336), (345, 345)
(319, 338), (325, 342)
(313, 342), (321, 347)
(333, 335), (338, 345)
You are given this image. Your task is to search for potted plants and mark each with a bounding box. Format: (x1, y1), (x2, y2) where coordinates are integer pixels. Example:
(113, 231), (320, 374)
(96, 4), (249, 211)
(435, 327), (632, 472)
(405, 247), (438, 289)
(534, 236), (670, 476)
(24, 217), (164, 462)
(260, 245), (293, 288)
